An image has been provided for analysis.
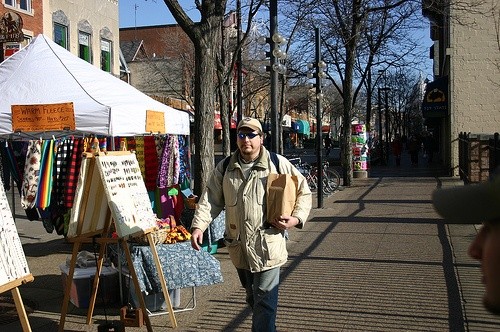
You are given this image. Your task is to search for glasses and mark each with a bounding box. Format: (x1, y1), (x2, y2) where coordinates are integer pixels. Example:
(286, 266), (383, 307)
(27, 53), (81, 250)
(238, 131), (260, 139)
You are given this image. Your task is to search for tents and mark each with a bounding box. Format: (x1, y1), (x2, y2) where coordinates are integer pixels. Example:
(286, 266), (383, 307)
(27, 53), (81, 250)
(0, 33), (191, 152)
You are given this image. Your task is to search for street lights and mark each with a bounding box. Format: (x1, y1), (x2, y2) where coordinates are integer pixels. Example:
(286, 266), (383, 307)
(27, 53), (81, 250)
(258, 34), (289, 154)
(377, 87), (391, 166)
(306, 28), (326, 210)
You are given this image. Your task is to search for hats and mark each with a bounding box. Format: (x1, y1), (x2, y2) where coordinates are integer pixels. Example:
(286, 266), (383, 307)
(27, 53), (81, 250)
(237, 117), (263, 136)
(432, 172), (500, 223)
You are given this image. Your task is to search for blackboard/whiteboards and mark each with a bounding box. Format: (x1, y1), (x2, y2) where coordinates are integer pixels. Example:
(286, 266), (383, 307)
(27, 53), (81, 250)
(66, 150), (158, 238)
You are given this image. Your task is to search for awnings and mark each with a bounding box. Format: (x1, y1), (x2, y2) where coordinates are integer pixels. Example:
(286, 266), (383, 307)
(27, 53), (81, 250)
(213, 114), (236, 129)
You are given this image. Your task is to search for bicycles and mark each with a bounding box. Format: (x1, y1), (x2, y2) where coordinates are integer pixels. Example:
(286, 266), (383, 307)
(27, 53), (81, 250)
(286, 155), (344, 195)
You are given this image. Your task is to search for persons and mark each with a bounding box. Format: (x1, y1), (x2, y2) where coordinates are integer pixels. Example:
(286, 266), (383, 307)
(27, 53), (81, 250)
(324, 134), (331, 148)
(190, 117), (313, 332)
(392, 133), (433, 169)
(432, 176), (500, 316)
